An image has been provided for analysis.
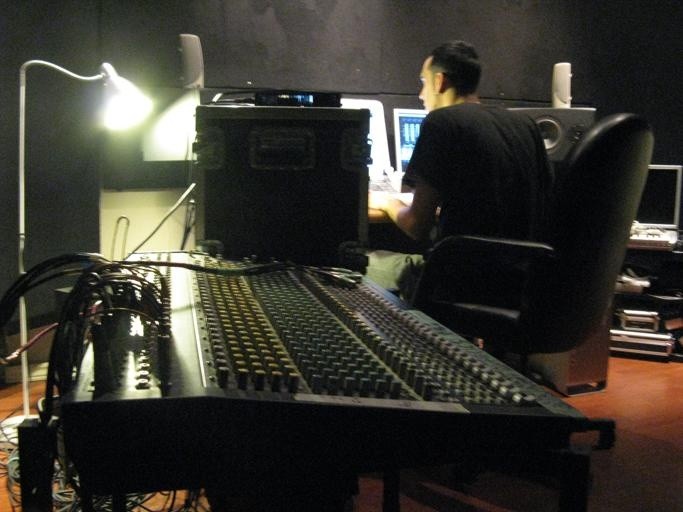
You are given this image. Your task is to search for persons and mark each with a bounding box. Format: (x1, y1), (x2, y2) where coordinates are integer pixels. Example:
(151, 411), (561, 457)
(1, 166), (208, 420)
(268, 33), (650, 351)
(366, 33), (553, 306)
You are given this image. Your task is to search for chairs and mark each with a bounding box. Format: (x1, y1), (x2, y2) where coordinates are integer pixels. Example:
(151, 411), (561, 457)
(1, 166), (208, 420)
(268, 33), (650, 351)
(408, 112), (655, 398)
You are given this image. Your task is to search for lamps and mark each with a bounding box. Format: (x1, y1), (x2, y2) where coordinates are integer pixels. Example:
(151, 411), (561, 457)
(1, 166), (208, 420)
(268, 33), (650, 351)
(0, 59), (155, 447)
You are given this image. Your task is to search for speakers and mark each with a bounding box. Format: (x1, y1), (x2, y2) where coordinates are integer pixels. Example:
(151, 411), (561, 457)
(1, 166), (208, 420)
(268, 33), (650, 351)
(506, 107), (595, 162)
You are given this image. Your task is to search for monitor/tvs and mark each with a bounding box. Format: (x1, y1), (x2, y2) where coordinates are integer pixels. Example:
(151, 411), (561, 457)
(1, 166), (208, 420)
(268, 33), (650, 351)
(393, 108), (429, 175)
(277, 95), (393, 183)
